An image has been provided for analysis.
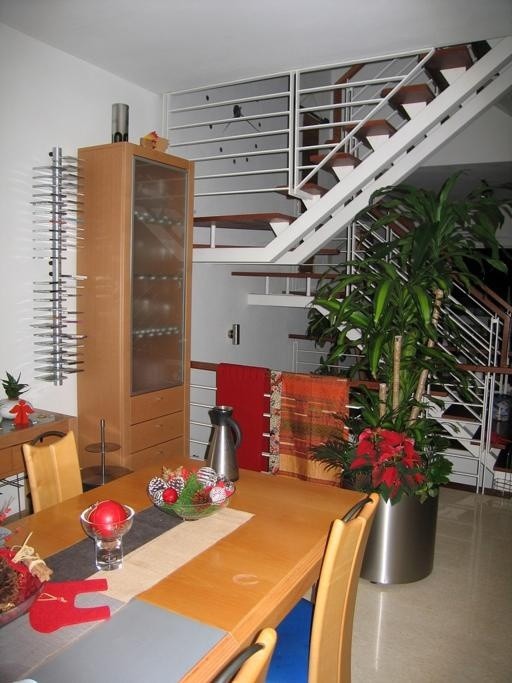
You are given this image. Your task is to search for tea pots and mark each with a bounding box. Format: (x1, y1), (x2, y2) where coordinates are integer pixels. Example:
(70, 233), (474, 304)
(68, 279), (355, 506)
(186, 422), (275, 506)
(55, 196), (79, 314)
(203, 406), (243, 484)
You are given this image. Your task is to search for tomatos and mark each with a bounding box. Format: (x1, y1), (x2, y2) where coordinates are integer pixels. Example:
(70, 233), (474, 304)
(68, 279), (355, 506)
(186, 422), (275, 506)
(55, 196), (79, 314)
(88, 500), (127, 536)
(163, 487), (177, 505)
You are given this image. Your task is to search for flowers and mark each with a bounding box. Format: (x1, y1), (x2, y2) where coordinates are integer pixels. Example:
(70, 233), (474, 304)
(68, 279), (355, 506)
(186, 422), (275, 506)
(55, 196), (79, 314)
(305, 387), (459, 507)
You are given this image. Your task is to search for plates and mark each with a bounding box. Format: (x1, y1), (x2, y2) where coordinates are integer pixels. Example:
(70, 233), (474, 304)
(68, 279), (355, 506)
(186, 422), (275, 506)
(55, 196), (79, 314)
(147, 477), (238, 521)
(0, 581), (45, 628)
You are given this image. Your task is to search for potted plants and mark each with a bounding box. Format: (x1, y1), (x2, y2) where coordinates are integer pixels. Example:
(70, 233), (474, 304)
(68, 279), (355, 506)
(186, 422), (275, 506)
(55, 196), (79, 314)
(0, 370), (33, 420)
(301, 161), (511, 588)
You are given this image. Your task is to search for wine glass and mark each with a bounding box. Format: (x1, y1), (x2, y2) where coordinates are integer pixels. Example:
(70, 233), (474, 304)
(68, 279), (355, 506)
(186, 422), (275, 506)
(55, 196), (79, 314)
(79, 503), (136, 573)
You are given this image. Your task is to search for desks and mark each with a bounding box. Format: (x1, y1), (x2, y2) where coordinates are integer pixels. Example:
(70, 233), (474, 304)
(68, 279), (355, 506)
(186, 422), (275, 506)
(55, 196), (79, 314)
(1, 408), (79, 500)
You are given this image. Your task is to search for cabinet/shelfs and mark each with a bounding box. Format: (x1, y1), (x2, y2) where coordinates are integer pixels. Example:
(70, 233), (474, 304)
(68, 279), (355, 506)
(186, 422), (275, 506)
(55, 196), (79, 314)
(75, 142), (196, 474)
